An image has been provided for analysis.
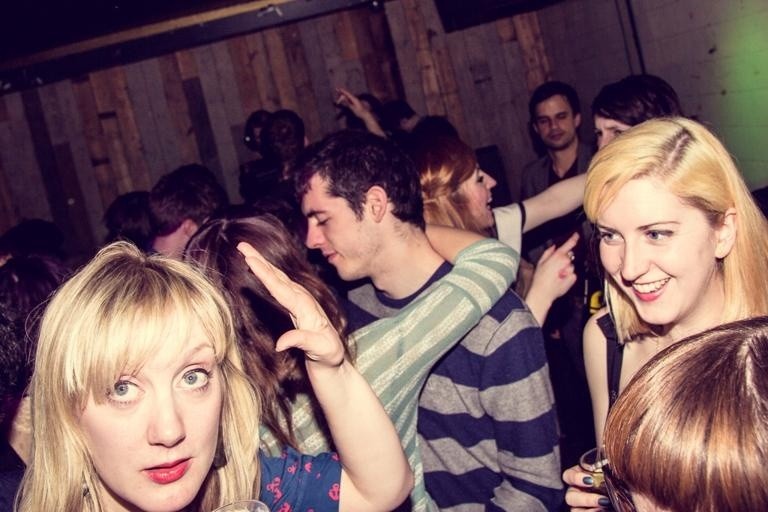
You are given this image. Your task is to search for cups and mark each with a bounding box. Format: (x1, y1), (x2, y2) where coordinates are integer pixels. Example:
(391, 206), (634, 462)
(577, 444), (615, 498)
